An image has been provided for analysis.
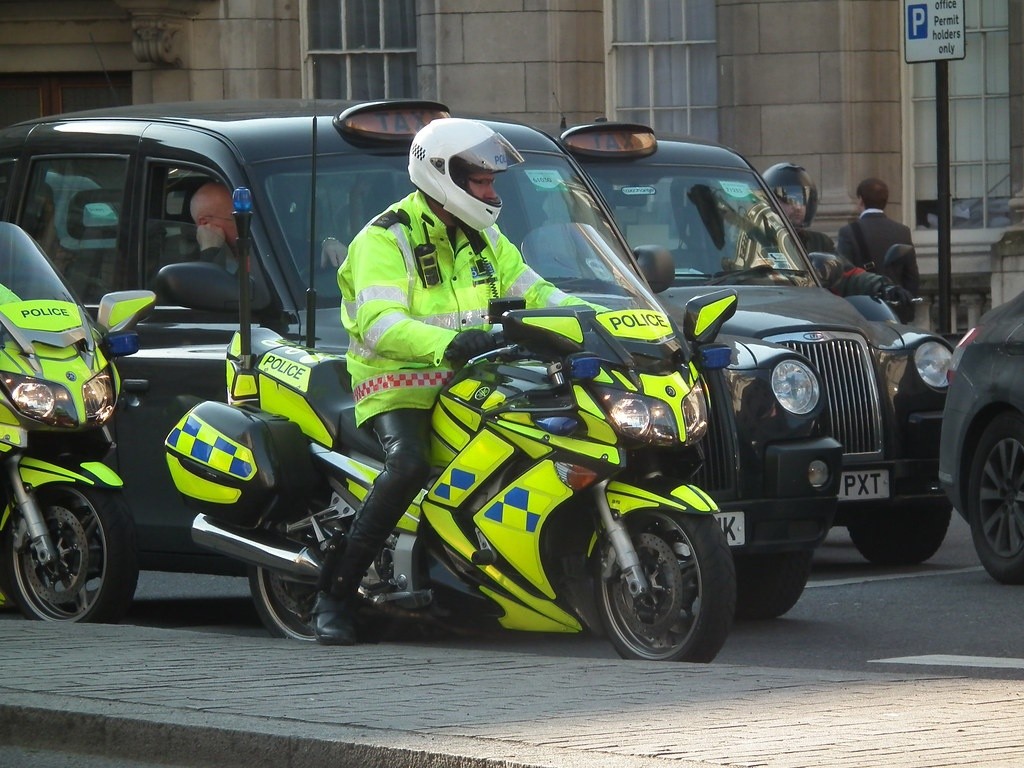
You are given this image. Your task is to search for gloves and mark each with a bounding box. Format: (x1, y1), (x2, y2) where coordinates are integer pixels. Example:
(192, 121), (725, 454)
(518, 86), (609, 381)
(879, 283), (916, 324)
(448, 329), (494, 359)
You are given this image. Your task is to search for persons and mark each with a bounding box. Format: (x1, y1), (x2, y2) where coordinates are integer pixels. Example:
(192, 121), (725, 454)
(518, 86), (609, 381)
(310, 116), (614, 647)
(836, 178), (921, 323)
(760, 162), (916, 325)
(180, 180), (349, 293)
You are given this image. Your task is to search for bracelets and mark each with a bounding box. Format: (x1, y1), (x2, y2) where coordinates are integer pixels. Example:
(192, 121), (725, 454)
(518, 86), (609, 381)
(321, 235), (339, 248)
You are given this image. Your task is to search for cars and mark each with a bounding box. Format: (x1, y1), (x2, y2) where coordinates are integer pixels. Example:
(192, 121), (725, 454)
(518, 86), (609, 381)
(938, 289), (1024, 583)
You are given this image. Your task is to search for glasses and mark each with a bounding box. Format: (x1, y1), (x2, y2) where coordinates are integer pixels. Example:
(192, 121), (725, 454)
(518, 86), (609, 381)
(205, 215), (234, 222)
(467, 176), (496, 185)
(779, 194), (810, 206)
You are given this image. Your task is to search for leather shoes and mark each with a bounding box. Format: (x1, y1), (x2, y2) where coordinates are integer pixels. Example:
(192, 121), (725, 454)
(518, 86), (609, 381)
(314, 594), (357, 645)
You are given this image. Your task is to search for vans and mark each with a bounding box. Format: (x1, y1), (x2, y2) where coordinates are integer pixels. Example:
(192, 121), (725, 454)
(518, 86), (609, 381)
(554, 115), (960, 565)
(1, 94), (846, 618)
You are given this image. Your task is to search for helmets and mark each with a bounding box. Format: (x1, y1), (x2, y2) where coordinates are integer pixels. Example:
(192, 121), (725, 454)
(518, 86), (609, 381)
(762, 162), (819, 226)
(408, 117), (524, 232)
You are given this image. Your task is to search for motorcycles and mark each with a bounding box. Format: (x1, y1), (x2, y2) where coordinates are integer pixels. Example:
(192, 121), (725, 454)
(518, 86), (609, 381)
(164, 188), (740, 663)
(1, 220), (156, 626)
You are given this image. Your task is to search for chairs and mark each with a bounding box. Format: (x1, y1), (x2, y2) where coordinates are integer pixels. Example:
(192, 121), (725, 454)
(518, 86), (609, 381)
(173, 178), (215, 257)
(19, 175), (176, 307)
